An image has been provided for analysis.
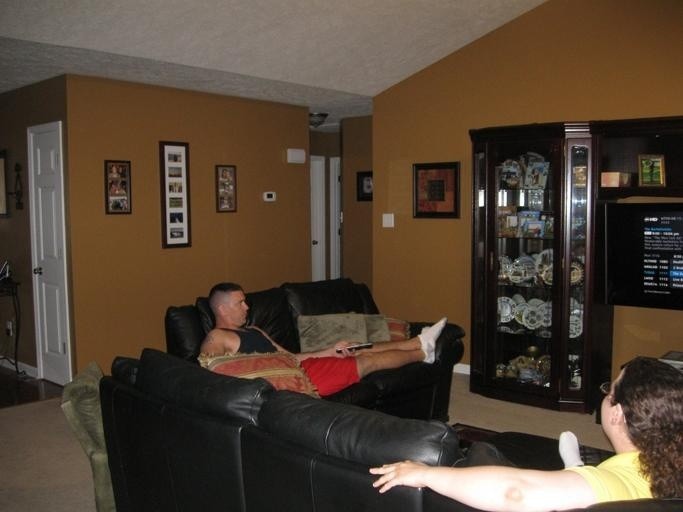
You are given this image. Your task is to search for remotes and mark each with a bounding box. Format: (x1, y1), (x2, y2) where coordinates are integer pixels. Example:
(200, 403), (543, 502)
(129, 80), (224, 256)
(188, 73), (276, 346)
(336, 342), (374, 354)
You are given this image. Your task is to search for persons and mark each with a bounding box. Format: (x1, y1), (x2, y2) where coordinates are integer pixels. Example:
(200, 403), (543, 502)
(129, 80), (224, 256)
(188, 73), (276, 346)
(226, 171), (233, 185)
(368, 354), (682, 511)
(106, 162), (129, 212)
(219, 183), (232, 197)
(219, 168), (228, 183)
(202, 281), (448, 404)
(220, 197), (232, 209)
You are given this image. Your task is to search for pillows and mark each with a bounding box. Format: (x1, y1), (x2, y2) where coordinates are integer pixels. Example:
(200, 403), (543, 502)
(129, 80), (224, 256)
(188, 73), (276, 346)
(385, 318), (411, 341)
(363, 313), (392, 342)
(199, 351), (322, 400)
(296, 314), (368, 352)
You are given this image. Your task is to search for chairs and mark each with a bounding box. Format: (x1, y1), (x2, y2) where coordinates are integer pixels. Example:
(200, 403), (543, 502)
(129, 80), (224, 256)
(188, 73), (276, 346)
(59, 360), (131, 512)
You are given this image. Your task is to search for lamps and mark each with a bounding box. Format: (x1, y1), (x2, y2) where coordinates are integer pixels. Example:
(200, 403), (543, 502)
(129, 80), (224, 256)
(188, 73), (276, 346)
(308, 113), (329, 128)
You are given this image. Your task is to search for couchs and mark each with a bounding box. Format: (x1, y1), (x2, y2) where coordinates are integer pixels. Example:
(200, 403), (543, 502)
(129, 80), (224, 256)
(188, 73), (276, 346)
(163, 278), (465, 425)
(101, 336), (683, 512)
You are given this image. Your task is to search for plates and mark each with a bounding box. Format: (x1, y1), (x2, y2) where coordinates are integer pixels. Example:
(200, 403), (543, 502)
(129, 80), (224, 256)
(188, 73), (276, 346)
(500, 159), (523, 186)
(537, 355), (552, 375)
(498, 294), (584, 338)
(498, 247), (585, 286)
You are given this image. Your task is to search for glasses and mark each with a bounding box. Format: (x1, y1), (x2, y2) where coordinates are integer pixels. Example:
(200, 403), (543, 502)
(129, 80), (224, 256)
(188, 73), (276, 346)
(599, 380), (619, 400)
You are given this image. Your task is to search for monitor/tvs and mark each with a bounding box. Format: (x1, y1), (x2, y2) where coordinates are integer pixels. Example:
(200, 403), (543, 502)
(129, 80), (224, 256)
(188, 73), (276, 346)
(604, 202), (683, 311)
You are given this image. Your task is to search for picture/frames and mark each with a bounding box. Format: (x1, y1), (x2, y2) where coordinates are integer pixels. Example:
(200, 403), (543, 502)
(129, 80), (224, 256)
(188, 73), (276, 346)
(524, 162), (549, 190)
(357, 170), (373, 202)
(412, 162), (461, 219)
(158, 141), (192, 248)
(104, 160), (132, 214)
(216, 165), (238, 213)
(639, 153), (667, 188)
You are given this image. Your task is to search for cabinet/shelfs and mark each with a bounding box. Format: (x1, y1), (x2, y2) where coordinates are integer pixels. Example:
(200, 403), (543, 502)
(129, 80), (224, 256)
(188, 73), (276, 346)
(469, 122), (613, 413)
